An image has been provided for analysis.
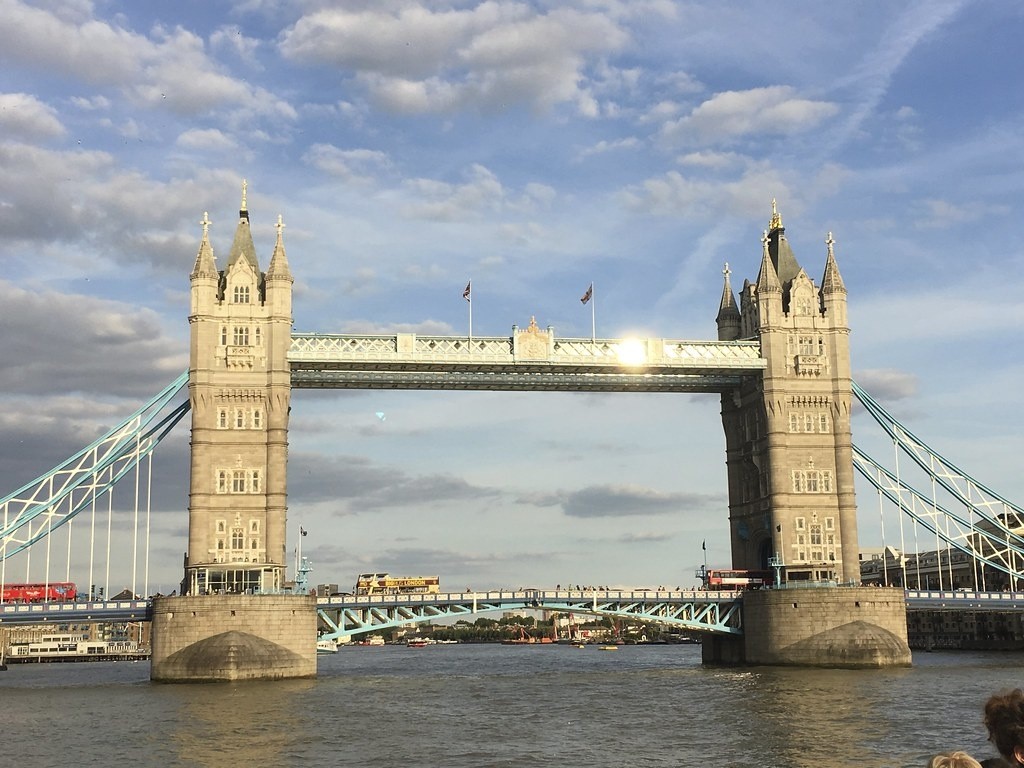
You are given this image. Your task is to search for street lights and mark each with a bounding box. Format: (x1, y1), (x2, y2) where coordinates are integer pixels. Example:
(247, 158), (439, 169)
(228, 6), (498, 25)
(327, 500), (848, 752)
(298, 524), (308, 570)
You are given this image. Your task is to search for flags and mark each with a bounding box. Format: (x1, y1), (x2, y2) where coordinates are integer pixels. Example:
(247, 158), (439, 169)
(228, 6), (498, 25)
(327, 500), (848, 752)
(580, 285), (593, 304)
(776, 525), (781, 532)
(703, 542), (706, 550)
(301, 527), (307, 536)
(463, 283), (470, 302)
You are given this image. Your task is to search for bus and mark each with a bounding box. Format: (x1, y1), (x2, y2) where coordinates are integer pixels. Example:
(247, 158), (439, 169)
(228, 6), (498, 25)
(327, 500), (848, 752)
(707, 568), (763, 590)
(356, 572), (439, 594)
(0, 582), (77, 603)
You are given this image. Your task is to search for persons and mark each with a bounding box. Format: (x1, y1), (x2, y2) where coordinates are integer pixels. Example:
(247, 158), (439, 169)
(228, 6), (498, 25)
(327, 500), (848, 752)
(500, 588), (503, 592)
(889, 582), (893, 587)
(1017, 588), (1024, 592)
(675, 586), (680, 591)
(569, 584), (610, 591)
(519, 587), (522, 591)
(994, 584), (1011, 592)
(915, 585), (919, 590)
(392, 588), (410, 594)
(692, 586), (706, 591)
(467, 588), (471, 592)
(861, 581), (878, 587)
(984, 689), (1024, 768)
(908, 585), (911, 590)
(928, 751), (1015, 768)
(658, 586), (665, 591)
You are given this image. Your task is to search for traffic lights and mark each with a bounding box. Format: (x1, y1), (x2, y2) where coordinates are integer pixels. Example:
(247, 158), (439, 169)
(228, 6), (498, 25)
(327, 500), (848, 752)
(91, 585), (94, 593)
(99, 588), (103, 595)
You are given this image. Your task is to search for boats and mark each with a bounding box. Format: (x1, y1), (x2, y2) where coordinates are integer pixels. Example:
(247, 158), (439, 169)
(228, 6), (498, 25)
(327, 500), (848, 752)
(599, 645), (619, 650)
(407, 640), (427, 647)
(442, 639), (457, 644)
(317, 641), (338, 654)
